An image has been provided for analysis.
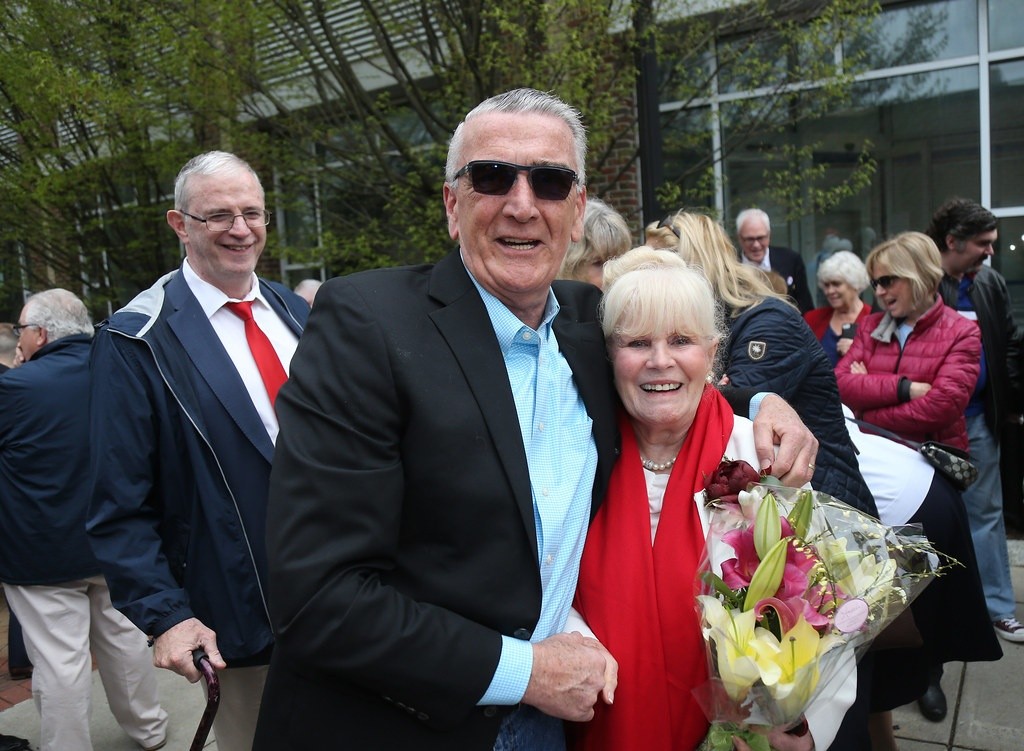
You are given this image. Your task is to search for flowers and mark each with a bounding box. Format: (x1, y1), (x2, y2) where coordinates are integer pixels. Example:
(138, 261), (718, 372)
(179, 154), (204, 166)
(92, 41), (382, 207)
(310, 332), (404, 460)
(688, 453), (967, 751)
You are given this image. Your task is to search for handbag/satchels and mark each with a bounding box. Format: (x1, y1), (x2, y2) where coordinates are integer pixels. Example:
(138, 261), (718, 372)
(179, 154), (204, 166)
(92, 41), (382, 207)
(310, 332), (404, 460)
(920, 441), (979, 487)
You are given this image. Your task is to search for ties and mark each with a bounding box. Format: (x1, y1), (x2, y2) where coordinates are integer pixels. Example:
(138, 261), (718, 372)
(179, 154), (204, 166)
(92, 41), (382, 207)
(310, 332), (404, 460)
(225, 301), (289, 409)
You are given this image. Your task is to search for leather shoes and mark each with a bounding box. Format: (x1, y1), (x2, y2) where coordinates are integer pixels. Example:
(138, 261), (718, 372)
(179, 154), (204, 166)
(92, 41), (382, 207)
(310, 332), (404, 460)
(918, 668), (947, 723)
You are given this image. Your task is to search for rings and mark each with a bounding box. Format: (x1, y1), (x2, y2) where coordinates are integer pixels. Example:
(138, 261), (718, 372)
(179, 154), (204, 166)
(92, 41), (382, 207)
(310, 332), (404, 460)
(808, 462), (816, 470)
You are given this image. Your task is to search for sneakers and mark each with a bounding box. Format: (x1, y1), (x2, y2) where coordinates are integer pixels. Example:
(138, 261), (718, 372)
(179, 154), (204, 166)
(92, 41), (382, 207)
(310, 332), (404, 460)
(993, 617), (1024, 642)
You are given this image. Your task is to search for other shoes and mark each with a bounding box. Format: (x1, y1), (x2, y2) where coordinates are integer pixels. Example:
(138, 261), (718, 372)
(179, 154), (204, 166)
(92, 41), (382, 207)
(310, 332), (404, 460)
(9, 666), (35, 680)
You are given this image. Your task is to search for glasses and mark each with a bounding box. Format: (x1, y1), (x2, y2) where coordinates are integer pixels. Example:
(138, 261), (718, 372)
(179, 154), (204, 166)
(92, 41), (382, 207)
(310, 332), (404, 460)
(870, 275), (899, 291)
(656, 214), (680, 239)
(453, 159), (578, 201)
(179, 209), (272, 232)
(740, 235), (767, 243)
(12, 324), (39, 337)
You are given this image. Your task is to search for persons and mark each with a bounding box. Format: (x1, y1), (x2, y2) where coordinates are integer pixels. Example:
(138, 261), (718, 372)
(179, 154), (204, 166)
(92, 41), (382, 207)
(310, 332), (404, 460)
(555, 196), (1024, 724)
(293, 278), (324, 309)
(0, 289), (171, 751)
(86, 151), (312, 751)
(267, 87), (819, 751)
(563, 246), (856, 751)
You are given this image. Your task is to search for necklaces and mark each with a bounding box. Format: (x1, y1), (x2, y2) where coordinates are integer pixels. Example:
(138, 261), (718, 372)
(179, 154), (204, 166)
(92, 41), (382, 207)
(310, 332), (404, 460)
(641, 455), (676, 471)
